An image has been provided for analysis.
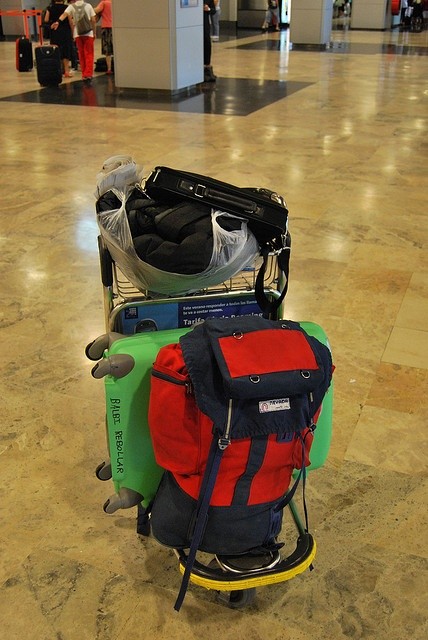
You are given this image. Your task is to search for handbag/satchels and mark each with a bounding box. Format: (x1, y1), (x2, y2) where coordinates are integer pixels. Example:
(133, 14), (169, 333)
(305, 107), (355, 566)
(41, 6), (52, 39)
(140, 166), (288, 246)
(94, 57), (114, 72)
(204, 66), (216, 82)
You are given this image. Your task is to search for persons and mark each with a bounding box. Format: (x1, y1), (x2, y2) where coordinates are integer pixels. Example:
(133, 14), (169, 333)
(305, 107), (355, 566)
(44, 0), (74, 78)
(208, 0), (221, 39)
(344, 0), (351, 17)
(94, 0), (114, 75)
(400, 0), (424, 28)
(262, 0), (280, 30)
(50, 0), (97, 83)
(203, 0), (216, 65)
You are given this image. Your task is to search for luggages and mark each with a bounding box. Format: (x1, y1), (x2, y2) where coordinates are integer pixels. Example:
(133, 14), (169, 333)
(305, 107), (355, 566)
(85, 322), (334, 514)
(35, 25), (62, 87)
(16, 35), (33, 72)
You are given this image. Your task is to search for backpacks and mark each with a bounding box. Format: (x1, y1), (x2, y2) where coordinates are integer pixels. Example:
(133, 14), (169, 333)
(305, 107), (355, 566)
(72, 2), (93, 35)
(136, 315), (335, 612)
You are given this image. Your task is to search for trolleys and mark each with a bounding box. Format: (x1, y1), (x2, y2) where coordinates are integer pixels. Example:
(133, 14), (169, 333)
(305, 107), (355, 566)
(98, 233), (317, 610)
